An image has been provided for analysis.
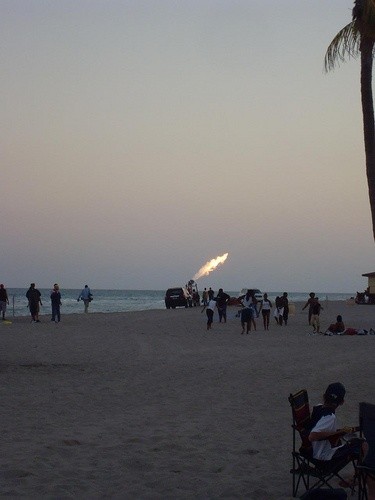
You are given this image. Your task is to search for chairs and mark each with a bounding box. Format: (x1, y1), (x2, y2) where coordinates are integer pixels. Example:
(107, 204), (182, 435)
(288, 389), (375, 500)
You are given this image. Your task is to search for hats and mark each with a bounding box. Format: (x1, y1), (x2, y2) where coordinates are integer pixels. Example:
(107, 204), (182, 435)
(327, 383), (345, 401)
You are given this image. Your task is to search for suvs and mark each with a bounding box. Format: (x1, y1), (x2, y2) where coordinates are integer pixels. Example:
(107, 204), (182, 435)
(239, 288), (264, 304)
(164, 287), (192, 309)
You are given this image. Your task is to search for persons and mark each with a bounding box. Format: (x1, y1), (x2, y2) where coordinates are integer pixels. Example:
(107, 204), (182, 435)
(300, 382), (375, 500)
(77, 285), (93, 313)
(187, 287), (345, 336)
(0, 283), (63, 324)
